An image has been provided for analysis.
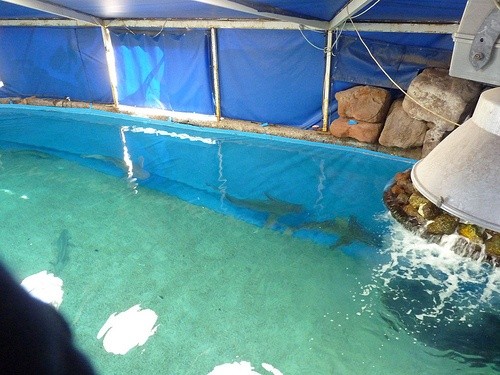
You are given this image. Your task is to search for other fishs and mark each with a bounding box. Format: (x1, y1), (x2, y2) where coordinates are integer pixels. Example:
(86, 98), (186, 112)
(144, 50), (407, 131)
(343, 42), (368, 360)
(223, 191), (303, 229)
(80, 154), (147, 179)
(10, 150), (49, 158)
(49, 230), (75, 276)
(280, 214), (383, 250)
